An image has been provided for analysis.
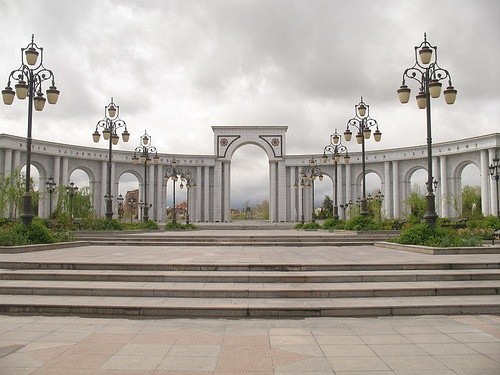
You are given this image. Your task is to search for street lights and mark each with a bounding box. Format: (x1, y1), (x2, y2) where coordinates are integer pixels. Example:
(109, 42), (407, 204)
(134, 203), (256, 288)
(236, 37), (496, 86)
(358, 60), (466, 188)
(138, 199), (145, 222)
(92, 96), (130, 220)
(321, 126), (349, 219)
(180, 168), (197, 224)
(132, 129), (159, 222)
(128, 197), (135, 222)
(344, 96), (382, 219)
(65, 180), (79, 226)
(116, 192), (124, 222)
(488, 154), (500, 217)
(375, 188), (385, 222)
(293, 174), (311, 224)
(299, 154), (323, 223)
(1, 34), (60, 232)
(45, 175), (56, 219)
(397, 31), (457, 227)
(163, 154), (185, 224)
